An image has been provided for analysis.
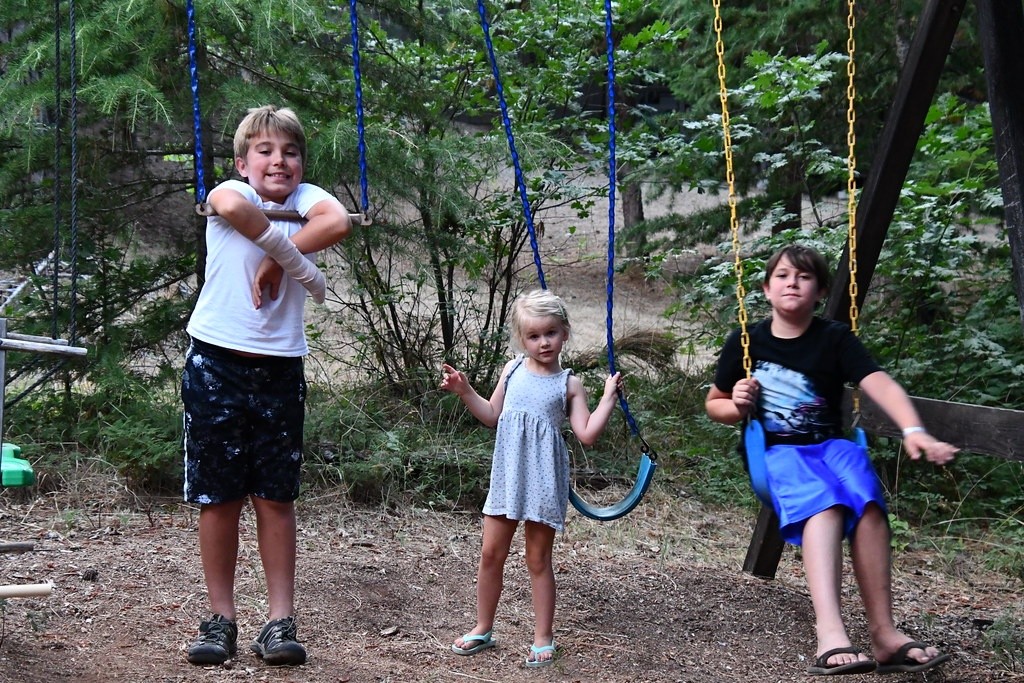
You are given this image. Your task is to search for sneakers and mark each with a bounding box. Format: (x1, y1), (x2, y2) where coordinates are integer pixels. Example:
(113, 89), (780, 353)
(187, 612), (238, 664)
(249, 615), (306, 665)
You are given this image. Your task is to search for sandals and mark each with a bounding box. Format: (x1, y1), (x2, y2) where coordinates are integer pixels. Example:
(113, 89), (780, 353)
(452, 628), (496, 656)
(525, 637), (555, 667)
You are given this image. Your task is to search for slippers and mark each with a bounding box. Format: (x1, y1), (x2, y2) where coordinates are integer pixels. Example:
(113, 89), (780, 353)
(806, 646), (877, 676)
(875, 641), (951, 674)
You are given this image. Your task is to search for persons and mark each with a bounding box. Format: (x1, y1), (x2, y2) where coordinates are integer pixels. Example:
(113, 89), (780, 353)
(180, 104), (353, 666)
(441, 290), (624, 669)
(704, 245), (961, 677)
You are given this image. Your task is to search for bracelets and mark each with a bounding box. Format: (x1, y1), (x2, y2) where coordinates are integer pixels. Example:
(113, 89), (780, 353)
(902, 426), (925, 436)
